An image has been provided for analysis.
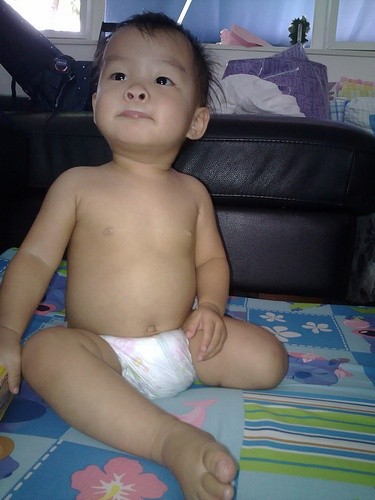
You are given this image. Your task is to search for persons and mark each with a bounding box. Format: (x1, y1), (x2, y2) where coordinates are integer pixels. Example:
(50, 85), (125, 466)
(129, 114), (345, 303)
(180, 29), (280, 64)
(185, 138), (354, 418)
(0, 10), (289, 500)
